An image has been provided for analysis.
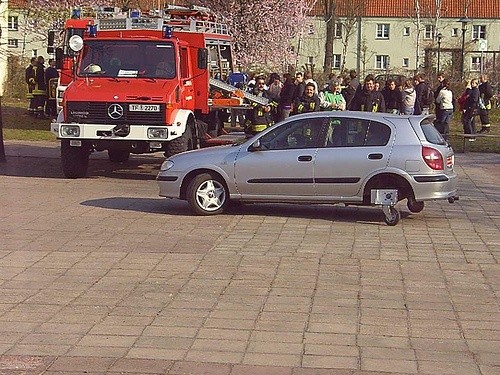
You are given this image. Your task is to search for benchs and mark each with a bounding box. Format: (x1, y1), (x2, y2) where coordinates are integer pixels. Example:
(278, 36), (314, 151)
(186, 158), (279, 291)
(442, 134), (500, 153)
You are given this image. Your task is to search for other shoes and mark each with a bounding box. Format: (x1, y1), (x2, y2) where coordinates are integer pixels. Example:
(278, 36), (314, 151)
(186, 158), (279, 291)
(477, 130), (482, 133)
(480, 130), (489, 134)
(469, 137), (476, 142)
(25, 109), (52, 120)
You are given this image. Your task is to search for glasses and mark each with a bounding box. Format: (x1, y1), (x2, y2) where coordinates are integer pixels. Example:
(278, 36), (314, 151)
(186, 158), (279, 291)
(258, 83), (265, 85)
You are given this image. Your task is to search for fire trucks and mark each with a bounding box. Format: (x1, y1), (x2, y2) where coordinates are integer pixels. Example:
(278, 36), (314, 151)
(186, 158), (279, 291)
(47, 5), (269, 178)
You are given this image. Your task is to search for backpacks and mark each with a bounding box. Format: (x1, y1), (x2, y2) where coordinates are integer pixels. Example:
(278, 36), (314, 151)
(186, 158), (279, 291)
(425, 86), (434, 104)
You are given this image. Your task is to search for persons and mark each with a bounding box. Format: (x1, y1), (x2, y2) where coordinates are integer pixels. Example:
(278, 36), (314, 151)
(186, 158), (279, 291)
(227, 71), (494, 143)
(27, 55), (58, 119)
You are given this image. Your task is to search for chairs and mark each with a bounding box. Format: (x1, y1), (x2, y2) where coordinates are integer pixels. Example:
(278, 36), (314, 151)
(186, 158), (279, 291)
(327, 124), (346, 146)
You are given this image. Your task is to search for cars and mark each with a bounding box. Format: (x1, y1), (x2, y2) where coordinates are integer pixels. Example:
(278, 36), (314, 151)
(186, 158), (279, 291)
(157, 112), (459, 225)
